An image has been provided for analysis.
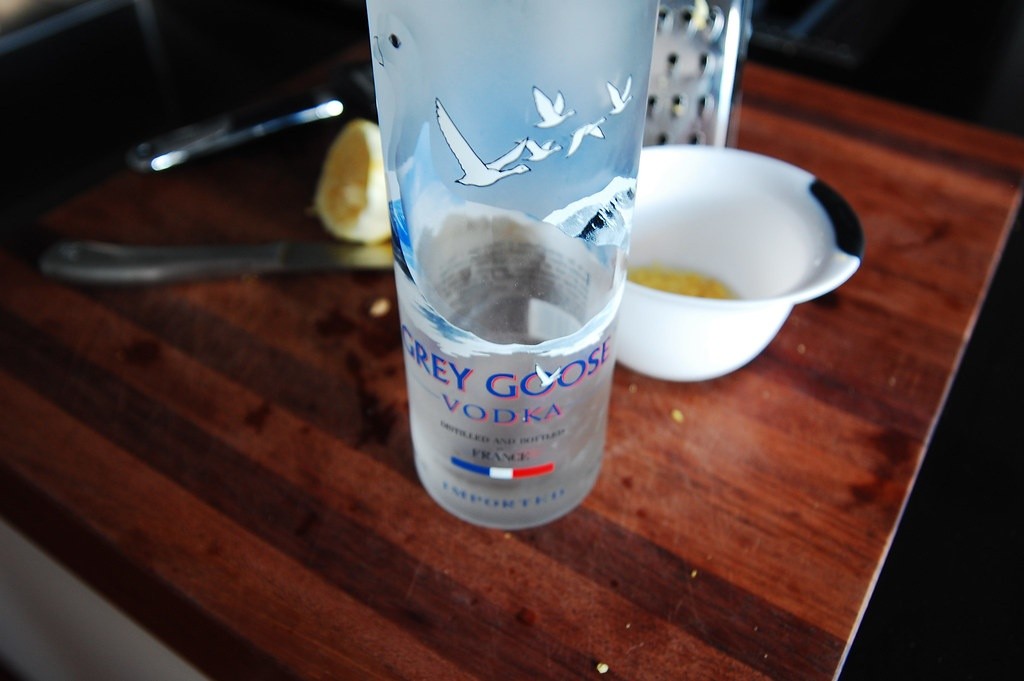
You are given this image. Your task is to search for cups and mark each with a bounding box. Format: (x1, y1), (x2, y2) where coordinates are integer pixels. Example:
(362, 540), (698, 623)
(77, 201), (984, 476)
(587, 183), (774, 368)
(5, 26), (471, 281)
(366, 0), (662, 528)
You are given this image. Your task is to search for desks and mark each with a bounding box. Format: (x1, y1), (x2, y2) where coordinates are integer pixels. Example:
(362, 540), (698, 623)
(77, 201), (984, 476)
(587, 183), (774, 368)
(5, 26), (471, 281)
(0, 40), (1024, 681)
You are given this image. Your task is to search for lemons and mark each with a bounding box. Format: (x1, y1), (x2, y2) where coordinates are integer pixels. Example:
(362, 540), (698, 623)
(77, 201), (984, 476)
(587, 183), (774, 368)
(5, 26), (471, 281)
(316, 120), (391, 245)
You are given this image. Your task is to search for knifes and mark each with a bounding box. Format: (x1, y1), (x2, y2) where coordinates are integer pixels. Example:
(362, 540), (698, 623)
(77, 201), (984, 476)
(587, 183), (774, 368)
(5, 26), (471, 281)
(35, 240), (394, 280)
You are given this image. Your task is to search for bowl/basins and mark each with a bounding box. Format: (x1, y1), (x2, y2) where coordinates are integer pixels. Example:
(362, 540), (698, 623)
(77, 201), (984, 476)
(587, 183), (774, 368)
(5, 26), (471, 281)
(616, 143), (866, 382)
